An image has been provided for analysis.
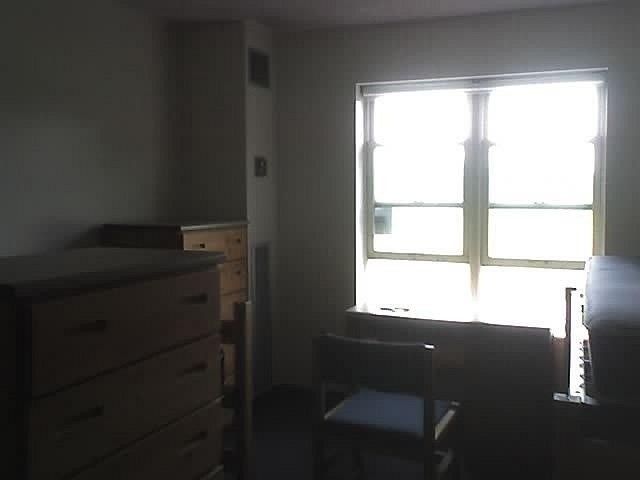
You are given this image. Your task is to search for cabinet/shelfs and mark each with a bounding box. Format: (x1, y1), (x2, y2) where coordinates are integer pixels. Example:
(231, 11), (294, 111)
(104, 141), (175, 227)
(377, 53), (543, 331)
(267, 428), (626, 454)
(107, 217), (248, 321)
(0, 247), (225, 480)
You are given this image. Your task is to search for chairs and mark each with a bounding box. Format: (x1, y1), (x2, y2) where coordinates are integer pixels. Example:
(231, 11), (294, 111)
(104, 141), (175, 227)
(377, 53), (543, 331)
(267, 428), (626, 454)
(310, 333), (461, 480)
